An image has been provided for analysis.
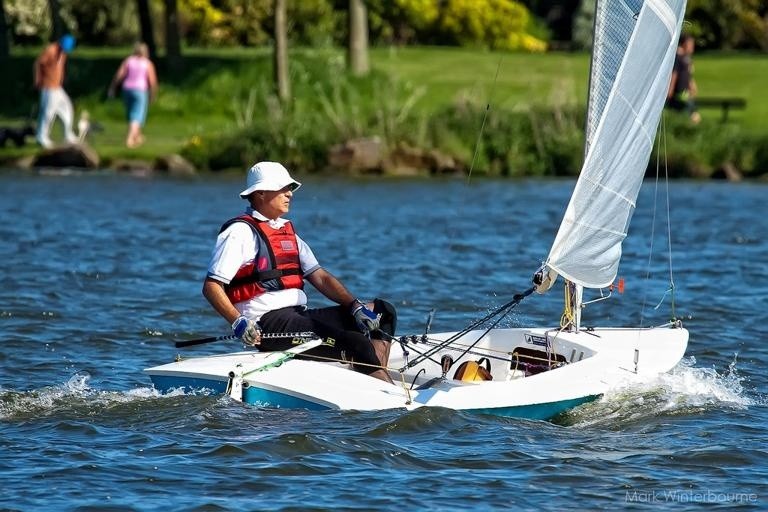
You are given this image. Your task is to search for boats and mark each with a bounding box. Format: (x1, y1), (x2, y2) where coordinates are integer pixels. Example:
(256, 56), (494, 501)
(142, 322), (690, 425)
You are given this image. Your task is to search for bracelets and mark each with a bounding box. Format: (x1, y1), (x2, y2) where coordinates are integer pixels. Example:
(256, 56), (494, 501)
(347, 297), (362, 312)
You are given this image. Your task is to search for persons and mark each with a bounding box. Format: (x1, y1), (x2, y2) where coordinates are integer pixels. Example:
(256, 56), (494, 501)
(30, 26), (80, 151)
(663, 33), (702, 124)
(106, 41), (162, 150)
(198, 158), (397, 385)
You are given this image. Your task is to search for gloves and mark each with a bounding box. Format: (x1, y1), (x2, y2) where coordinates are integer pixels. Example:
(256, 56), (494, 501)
(231, 315), (262, 346)
(347, 298), (379, 330)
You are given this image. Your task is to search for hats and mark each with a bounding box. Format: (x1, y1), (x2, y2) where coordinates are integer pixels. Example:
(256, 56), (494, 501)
(239, 162), (302, 200)
(60, 34), (74, 53)
